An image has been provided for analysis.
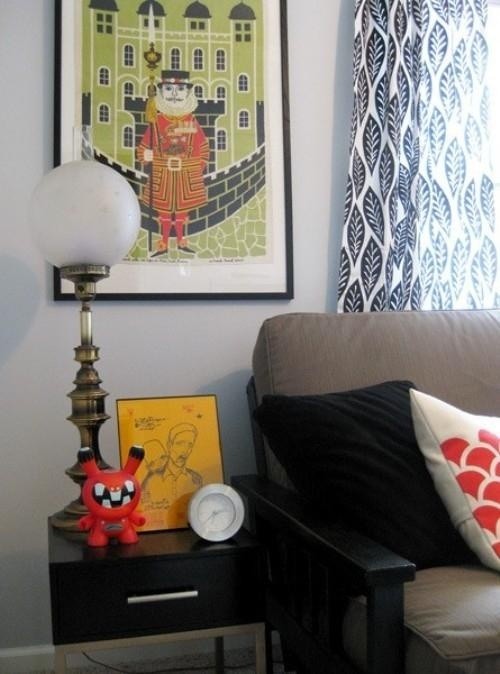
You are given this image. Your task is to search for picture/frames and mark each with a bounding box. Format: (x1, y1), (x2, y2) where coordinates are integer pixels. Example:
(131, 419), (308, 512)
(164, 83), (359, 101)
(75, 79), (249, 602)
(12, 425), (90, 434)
(51, 1), (291, 296)
(114, 394), (226, 537)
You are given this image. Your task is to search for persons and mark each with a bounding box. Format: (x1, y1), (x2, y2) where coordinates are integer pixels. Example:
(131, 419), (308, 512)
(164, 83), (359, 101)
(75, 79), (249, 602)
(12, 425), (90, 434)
(140, 423), (203, 509)
(136, 69), (210, 258)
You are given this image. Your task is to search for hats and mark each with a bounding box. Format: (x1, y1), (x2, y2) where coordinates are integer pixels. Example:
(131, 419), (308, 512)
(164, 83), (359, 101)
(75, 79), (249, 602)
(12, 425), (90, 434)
(157, 70), (193, 89)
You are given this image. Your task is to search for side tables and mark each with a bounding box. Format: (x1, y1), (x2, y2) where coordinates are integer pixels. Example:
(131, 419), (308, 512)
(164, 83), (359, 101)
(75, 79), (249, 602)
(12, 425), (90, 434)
(46, 499), (280, 670)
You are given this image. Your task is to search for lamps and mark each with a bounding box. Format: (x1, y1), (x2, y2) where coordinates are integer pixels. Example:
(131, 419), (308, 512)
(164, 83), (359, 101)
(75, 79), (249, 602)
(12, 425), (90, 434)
(39, 156), (140, 533)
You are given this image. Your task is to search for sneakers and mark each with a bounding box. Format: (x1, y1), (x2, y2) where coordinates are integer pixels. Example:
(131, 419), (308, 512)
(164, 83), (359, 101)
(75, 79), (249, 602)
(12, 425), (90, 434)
(150, 248), (168, 258)
(177, 244), (196, 255)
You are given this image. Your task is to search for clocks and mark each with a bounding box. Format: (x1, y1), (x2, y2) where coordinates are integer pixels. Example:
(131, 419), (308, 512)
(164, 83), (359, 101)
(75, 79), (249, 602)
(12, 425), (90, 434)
(186, 485), (244, 542)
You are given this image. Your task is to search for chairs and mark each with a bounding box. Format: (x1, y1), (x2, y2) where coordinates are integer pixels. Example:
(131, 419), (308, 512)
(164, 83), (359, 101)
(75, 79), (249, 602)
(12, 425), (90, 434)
(233, 307), (498, 674)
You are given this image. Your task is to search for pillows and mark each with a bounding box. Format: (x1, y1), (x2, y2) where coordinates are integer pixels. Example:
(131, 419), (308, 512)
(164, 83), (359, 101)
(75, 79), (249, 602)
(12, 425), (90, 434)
(411, 388), (499, 570)
(255, 379), (480, 567)
